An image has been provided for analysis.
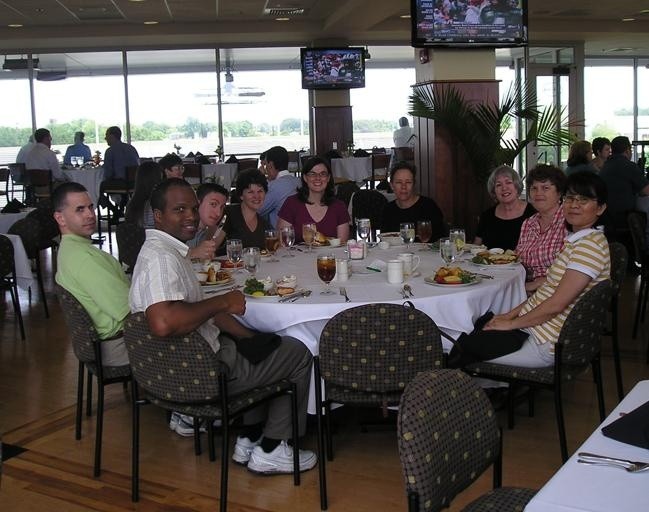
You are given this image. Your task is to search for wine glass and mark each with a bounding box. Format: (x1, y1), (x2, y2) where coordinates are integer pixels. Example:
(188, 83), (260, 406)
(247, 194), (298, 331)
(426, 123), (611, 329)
(450, 230), (465, 257)
(302, 224), (316, 254)
(265, 230), (280, 262)
(317, 255), (337, 296)
(418, 221), (432, 251)
(400, 223), (416, 254)
(71, 156), (77, 168)
(440, 238), (457, 269)
(358, 220), (370, 251)
(280, 227), (295, 257)
(226, 239), (243, 273)
(243, 247), (260, 281)
(76, 156), (84, 170)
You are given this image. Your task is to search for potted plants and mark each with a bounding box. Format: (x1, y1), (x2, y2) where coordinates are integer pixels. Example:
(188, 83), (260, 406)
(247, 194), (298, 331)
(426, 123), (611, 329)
(214, 145), (224, 165)
(92, 151), (102, 164)
(174, 143), (182, 156)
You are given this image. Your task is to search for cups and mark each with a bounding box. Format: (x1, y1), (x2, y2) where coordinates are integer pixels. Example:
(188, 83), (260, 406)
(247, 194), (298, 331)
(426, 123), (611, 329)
(398, 254), (420, 276)
(347, 242), (365, 260)
(388, 260), (404, 284)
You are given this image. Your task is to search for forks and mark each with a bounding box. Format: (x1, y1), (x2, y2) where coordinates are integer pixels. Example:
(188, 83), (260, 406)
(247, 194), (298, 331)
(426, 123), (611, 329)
(577, 456), (649, 474)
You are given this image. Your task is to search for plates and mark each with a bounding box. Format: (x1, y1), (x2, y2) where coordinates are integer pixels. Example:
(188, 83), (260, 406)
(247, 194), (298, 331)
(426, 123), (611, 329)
(241, 285), (297, 300)
(470, 261), (511, 266)
(464, 244), (476, 251)
(197, 283), (238, 292)
(424, 275), (480, 286)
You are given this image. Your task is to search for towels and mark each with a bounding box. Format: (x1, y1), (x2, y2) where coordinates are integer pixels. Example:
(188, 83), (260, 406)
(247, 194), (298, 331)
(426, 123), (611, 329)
(601, 399), (648, 450)
(447, 310), (531, 363)
(238, 331), (282, 364)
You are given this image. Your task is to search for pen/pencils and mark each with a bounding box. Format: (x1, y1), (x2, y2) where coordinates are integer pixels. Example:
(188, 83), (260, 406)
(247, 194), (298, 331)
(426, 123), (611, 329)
(366, 266), (381, 272)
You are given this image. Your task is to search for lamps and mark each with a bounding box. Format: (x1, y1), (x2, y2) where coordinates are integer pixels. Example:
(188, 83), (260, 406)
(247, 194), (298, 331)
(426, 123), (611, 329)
(225, 69), (233, 83)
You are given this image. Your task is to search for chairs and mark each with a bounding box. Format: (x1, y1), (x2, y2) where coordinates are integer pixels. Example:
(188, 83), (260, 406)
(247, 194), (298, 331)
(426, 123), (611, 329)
(300, 156), (313, 168)
(155, 157), (164, 164)
(324, 149), (343, 159)
(139, 158), (152, 166)
(397, 369), (541, 512)
(230, 158), (258, 187)
(8, 163), (25, 203)
(0, 164), (11, 203)
(53, 275), (202, 478)
(6, 218), (50, 319)
(627, 211), (649, 339)
(373, 146), (386, 154)
(362, 154), (391, 190)
(124, 310), (300, 511)
(26, 208), (61, 251)
(287, 152), (300, 178)
(608, 242), (627, 403)
(354, 148), (370, 157)
(314, 302), (444, 511)
(352, 189), (388, 239)
(461, 278), (612, 465)
(183, 163), (201, 195)
(337, 182), (359, 207)
(0, 234), (26, 343)
(97, 166), (139, 232)
(23, 169), (53, 206)
(182, 158), (195, 165)
(116, 222), (146, 274)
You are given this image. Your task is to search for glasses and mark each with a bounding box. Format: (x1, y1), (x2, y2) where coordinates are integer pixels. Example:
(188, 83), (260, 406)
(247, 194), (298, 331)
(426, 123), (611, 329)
(564, 193), (600, 205)
(306, 171), (330, 177)
(171, 164), (187, 172)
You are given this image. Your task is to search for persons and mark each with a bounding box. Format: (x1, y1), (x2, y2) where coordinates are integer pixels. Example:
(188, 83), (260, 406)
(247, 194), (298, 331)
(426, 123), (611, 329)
(591, 138), (610, 172)
(99, 126), (140, 225)
(278, 156), (352, 241)
(144, 153), (185, 231)
(24, 129), (65, 194)
(187, 181), (226, 259)
(372, 162), (444, 244)
(64, 131), (91, 167)
(393, 117), (415, 148)
(219, 168), (273, 254)
(16, 134), (36, 169)
(258, 146), (302, 230)
(512, 165), (568, 296)
(563, 140), (602, 177)
(476, 164), (537, 252)
(480, 173), (612, 368)
(131, 177), (319, 475)
(598, 136), (649, 228)
(49, 181), (233, 438)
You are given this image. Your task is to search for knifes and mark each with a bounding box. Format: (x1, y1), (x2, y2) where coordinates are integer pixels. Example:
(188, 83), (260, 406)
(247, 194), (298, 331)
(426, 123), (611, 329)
(579, 452), (649, 467)
(210, 214), (227, 242)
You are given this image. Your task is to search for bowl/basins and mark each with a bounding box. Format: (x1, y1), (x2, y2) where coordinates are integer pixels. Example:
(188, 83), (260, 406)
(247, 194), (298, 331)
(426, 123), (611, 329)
(470, 246), (487, 255)
(378, 232), (401, 246)
(329, 239), (341, 246)
(380, 242), (390, 249)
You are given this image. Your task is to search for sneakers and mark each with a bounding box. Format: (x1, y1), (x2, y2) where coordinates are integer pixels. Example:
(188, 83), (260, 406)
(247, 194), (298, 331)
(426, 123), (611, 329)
(175, 416), (216, 437)
(246, 440), (319, 474)
(167, 411), (185, 431)
(230, 433), (265, 464)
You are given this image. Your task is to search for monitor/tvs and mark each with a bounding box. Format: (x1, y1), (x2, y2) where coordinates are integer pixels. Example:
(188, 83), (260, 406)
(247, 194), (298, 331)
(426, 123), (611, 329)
(409, 0), (530, 47)
(301, 47), (367, 88)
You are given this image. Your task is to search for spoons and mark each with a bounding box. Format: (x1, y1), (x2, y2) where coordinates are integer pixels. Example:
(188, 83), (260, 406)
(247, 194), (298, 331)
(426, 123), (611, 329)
(292, 291), (312, 302)
(404, 285), (414, 296)
(340, 287), (351, 303)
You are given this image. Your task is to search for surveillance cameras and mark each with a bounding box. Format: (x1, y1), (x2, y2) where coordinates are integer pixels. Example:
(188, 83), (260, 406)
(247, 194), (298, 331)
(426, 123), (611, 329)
(224, 66), (233, 82)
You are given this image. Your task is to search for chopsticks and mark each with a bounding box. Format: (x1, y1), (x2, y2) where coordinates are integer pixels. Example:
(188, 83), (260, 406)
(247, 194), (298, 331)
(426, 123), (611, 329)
(474, 272), (493, 280)
(399, 288), (408, 298)
